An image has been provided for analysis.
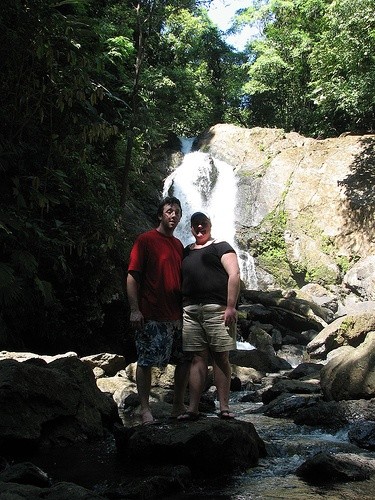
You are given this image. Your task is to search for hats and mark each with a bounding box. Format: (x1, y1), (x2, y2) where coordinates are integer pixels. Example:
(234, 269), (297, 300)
(191, 209), (210, 221)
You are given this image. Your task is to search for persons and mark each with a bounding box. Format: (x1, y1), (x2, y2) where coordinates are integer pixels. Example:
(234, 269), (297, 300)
(175, 212), (240, 421)
(125, 196), (208, 428)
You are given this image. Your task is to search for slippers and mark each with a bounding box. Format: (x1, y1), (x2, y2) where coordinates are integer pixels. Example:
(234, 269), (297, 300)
(169, 411), (207, 420)
(177, 412), (201, 421)
(217, 410), (235, 420)
(143, 418), (162, 427)
